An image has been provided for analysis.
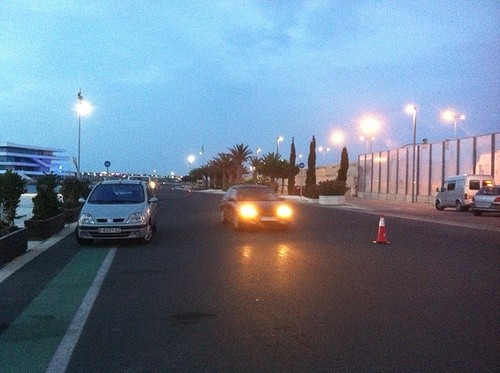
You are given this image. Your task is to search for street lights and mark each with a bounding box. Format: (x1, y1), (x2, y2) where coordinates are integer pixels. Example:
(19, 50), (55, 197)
(407, 105), (418, 144)
(276, 136), (283, 154)
(256, 148), (261, 160)
(331, 131), (344, 164)
(74, 87), (91, 172)
(362, 117), (376, 153)
(444, 111), (465, 140)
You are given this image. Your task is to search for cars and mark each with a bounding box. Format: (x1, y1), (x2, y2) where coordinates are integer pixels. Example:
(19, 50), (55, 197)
(75, 179), (159, 245)
(128, 175), (217, 192)
(470, 185), (500, 217)
(219, 184), (298, 233)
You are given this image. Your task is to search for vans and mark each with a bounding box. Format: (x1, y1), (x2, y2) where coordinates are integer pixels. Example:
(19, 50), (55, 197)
(435, 174), (496, 213)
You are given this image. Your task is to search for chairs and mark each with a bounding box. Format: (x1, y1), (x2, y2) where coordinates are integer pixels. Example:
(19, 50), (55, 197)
(132, 188), (144, 202)
(95, 187), (115, 202)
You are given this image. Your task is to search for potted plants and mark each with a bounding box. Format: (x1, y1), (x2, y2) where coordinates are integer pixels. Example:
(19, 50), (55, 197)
(24, 174), (66, 240)
(58, 178), (86, 223)
(0, 170), (28, 266)
(318, 180), (351, 204)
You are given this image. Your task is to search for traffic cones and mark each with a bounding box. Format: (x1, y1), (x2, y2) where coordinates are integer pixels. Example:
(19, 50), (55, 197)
(372, 214), (392, 244)
